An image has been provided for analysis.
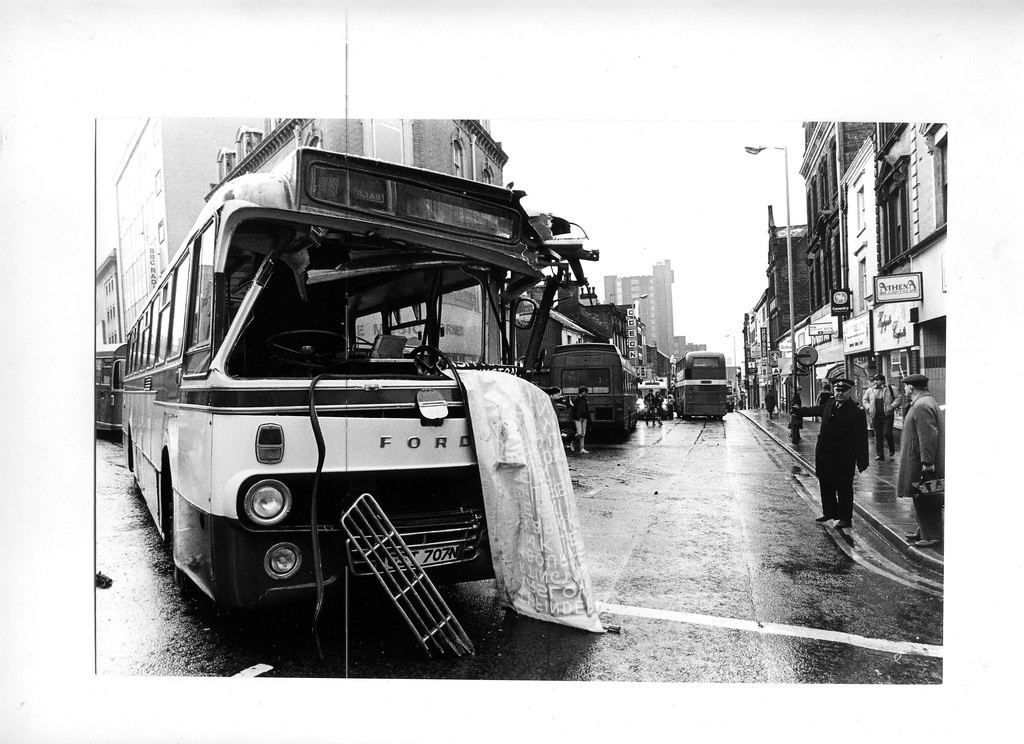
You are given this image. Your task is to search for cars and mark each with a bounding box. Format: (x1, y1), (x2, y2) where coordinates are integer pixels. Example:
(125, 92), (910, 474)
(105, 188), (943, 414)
(726, 382), (747, 412)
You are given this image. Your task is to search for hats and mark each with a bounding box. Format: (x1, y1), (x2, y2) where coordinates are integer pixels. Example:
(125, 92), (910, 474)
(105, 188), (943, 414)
(873, 374), (885, 380)
(900, 373), (930, 386)
(831, 378), (854, 389)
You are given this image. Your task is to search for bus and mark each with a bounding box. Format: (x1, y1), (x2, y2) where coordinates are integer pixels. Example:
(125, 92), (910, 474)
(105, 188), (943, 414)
(675, 351), (726, 419)
(637, 380), (671, 420)
(553, 343), (643, 442)
(96, 341), (128, 438)
(123, 147), (551, 613)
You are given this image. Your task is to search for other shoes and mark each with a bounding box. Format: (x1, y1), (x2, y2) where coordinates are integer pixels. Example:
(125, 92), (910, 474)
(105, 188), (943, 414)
(816, 513), (852, 530)
(905, 528), (940, 548)
(874, 448), (895, 460)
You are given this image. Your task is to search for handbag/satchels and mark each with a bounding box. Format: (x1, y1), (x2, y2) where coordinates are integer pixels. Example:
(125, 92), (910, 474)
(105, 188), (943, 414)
(913, 469), (944, 512)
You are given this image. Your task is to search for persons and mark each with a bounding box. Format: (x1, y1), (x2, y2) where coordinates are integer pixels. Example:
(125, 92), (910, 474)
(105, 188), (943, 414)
(862, 374), (903, 460)
(655, 390), (664, 425)
(897, 374), (944, 549)
(792, 378), (870, 527)
(570, 386), (590, 454)
(791, 386), (803, 444)
(815, 384), (834, 406)
(765, 389), (776, 419)
(644, 389), (655, 426)
(549, 386), (562, 420)
(667, 391), (675, 420)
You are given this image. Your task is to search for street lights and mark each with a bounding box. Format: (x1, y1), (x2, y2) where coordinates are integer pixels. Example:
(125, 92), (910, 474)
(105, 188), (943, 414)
(744, 143), (799, 396)
(726, 334), (739, 411)
(631, 294), (648, 368)
